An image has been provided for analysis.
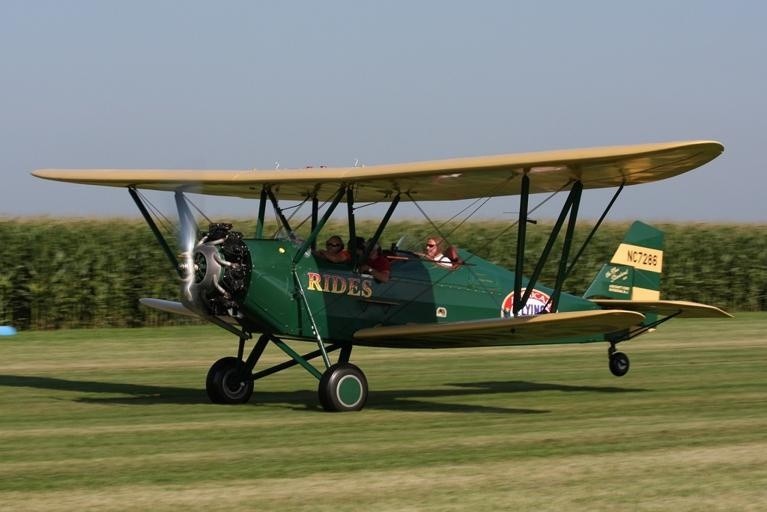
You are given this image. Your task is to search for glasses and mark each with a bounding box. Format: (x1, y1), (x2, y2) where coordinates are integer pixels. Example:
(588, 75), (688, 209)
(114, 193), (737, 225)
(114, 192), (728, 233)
(327, 243), (339, 247)
(427, 244), (433, 247)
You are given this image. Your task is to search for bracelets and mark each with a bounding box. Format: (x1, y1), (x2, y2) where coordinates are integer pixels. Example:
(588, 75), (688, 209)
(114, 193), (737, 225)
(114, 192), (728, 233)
(367, 268), (373, 273)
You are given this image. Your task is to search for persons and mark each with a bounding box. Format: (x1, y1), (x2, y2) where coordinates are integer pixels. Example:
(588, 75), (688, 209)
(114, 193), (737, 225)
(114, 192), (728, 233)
(413, 236), (453, 266)
(360, 238), (391, 283)
(316, 236), (352, 264)
(348, 237), (366, 259)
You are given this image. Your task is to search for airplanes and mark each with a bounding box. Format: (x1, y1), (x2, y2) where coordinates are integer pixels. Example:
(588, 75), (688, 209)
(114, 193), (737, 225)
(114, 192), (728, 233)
(29, 140), (733, 413)
